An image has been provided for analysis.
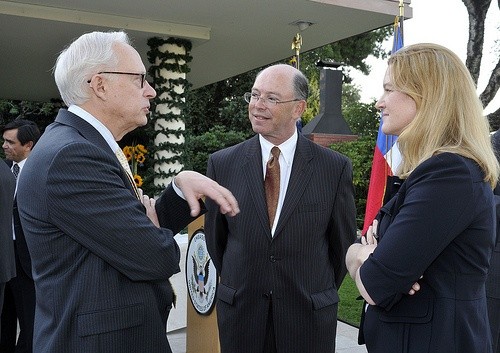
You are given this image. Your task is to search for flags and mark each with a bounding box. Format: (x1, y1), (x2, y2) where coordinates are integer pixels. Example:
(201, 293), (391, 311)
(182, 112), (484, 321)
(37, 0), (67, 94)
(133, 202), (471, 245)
(360, 17), (403, 234)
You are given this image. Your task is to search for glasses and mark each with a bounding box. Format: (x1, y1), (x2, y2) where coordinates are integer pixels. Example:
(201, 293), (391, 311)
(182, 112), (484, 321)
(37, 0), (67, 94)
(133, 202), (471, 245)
(87, 71), (146, 88)
(244, 92), (301, 108)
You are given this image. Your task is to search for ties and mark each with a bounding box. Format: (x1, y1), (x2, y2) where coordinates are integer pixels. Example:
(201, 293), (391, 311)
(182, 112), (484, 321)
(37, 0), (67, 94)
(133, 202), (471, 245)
(12, 164), (20, 177)
(264, 146), (281, 231)
(115, 146), (178, 309)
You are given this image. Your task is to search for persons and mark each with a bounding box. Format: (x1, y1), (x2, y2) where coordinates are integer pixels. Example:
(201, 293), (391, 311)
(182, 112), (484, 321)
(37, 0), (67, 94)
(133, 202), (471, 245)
(16, 31), (240, 353)
(205, 64), (358, 353)
(0, 157), (19, 353)
(2, 119), (40, 353)
(344, 43), (500, 353)
